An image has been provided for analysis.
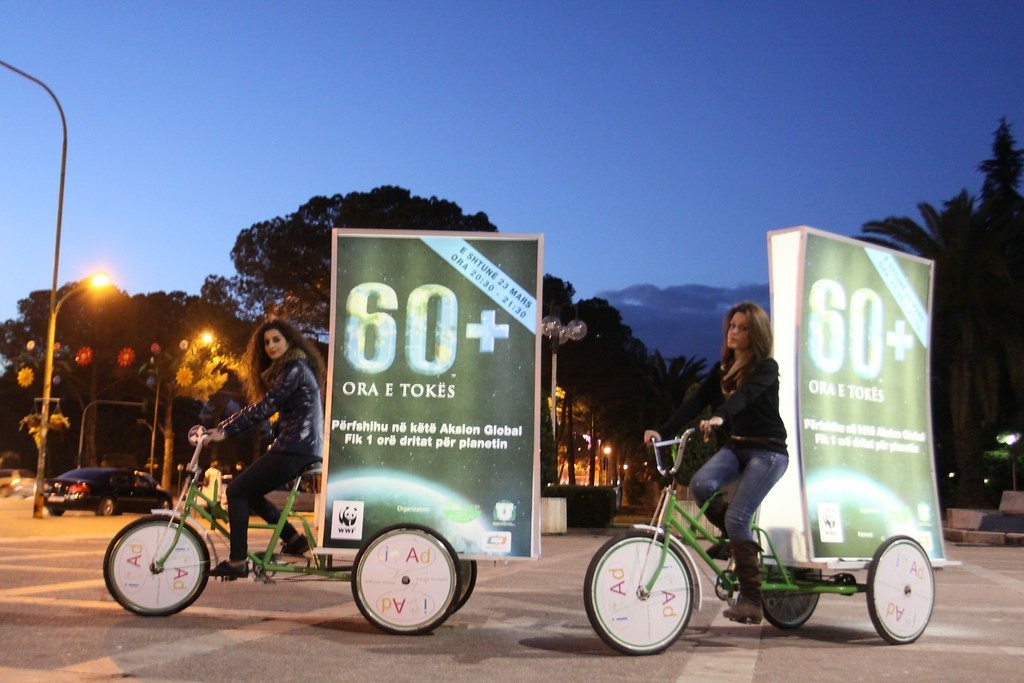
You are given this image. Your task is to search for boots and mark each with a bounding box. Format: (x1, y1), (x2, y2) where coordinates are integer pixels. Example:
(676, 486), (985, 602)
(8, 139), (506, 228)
(695, 494), (733, 560)
(722, 536), (764, 622)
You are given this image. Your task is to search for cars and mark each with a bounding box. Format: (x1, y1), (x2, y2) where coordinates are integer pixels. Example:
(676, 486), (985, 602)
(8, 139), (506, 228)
(0, 468), (38, 498)
(45, 463), (175, 518)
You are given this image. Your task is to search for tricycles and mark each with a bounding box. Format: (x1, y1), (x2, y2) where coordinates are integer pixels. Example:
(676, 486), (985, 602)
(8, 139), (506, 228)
(97, 226), (550, 635)
(582, 222), (964, 657)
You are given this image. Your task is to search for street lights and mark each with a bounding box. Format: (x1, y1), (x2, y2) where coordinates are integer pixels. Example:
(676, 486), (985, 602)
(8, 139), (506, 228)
(603, 445), (613, 487)
(541, 315), (589, 488)
(0, 58), (111, 519)
(995, 430), (1022, 492)
(136, 331), (215, 477)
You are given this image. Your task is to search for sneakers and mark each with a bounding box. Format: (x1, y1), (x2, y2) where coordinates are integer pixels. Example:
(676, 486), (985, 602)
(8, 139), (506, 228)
(209, 560), (250, 579)
(278, 533), (310, 555)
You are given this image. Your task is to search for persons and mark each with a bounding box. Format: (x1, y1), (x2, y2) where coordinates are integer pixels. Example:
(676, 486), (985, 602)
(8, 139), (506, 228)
(643, 301), (790, 626)
(201, 459), (223, 518)
(196, 317), (326, 580)
(220, 460), (247, 483)
